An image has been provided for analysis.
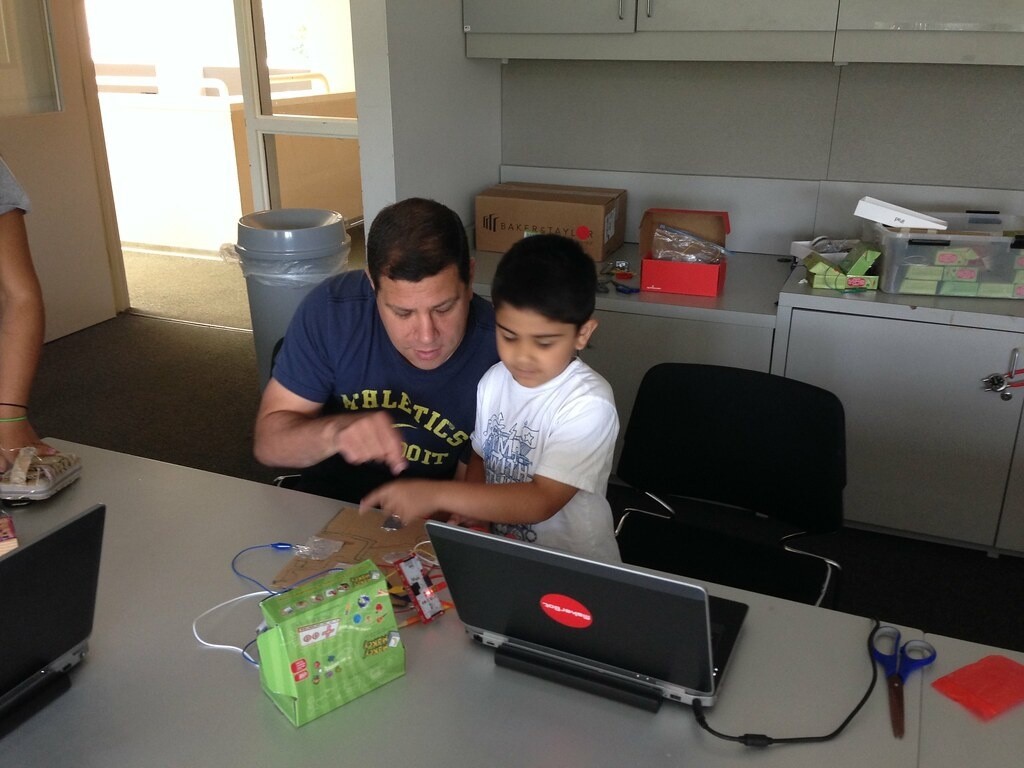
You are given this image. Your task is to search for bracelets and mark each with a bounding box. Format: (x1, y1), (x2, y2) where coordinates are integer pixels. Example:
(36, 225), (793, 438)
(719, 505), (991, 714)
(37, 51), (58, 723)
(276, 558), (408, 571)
(0, 415), (28, 422)
(0, 402), (28, 409)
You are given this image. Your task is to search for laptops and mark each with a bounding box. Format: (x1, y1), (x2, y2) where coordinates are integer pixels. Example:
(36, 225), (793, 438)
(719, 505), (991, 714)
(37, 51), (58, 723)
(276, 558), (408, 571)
(422, 518), (749, 714)
(0, 503), (108, 741)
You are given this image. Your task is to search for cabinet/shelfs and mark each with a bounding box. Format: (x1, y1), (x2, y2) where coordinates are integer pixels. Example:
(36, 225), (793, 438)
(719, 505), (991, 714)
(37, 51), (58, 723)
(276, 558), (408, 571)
(461, 226), (781, 489)
(771, 269), (1023, 561)
(460, 0), (1024, 70)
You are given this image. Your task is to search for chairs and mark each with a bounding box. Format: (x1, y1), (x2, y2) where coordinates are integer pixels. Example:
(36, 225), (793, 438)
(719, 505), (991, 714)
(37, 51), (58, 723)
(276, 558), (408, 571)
(596, 360), (848, 610)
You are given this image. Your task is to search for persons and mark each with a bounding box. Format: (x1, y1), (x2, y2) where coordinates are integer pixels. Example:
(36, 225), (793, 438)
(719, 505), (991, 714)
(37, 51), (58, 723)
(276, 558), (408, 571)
(0, 155), (58, 476)
(253, 196), (496, 522)
(357, 232), (624, 567)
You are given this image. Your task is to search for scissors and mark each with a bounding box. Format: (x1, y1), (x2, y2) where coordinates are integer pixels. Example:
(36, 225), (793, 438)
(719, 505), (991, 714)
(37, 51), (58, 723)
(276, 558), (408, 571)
(610, 278), (640, 294)
(867, 626), (936, 738)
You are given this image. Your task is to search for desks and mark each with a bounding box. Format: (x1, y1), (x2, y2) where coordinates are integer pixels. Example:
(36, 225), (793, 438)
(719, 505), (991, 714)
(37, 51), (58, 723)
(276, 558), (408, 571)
(0, 435), (1024, 768)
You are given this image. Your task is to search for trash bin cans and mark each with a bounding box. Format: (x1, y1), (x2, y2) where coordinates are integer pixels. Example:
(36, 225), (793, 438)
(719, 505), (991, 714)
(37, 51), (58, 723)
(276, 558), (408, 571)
(235, 208), (352, 395)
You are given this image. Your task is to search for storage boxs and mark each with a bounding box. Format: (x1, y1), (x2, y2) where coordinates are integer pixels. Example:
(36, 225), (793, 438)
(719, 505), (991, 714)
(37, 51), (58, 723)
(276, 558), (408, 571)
(789, 196), (1024, 299)
(474, 182), (627, 263)
(257, 561), (407, 729)
(637, 207), (731, 297)
